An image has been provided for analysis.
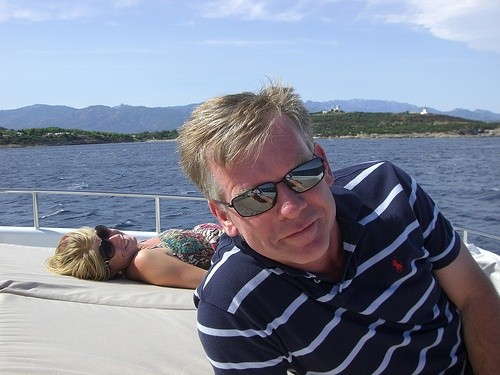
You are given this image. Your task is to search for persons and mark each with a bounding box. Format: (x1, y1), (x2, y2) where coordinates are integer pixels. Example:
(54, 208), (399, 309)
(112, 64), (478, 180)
(176, 77), (500, 375)
(48, 223), (225, 289)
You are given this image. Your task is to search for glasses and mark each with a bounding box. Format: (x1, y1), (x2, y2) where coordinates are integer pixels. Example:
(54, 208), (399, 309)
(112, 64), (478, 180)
(213, 154), (326, 217)
(95, 225), (115, 268)
